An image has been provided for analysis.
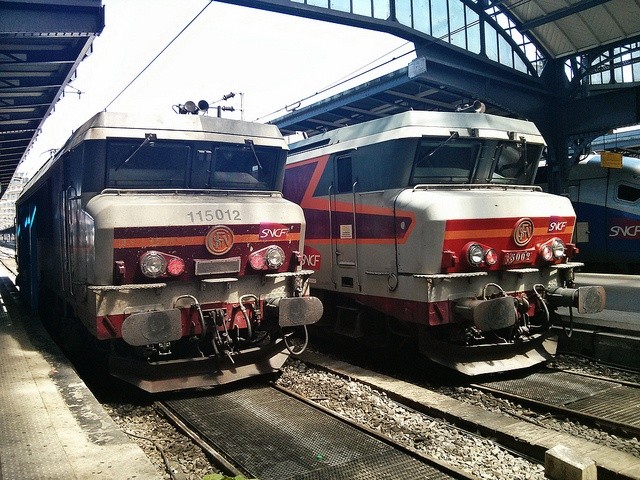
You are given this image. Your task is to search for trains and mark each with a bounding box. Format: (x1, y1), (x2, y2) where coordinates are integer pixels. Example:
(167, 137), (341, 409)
(285, 101), (607, 383)
(537, 154), (640, 270)
(12, 108), (323, 397)
